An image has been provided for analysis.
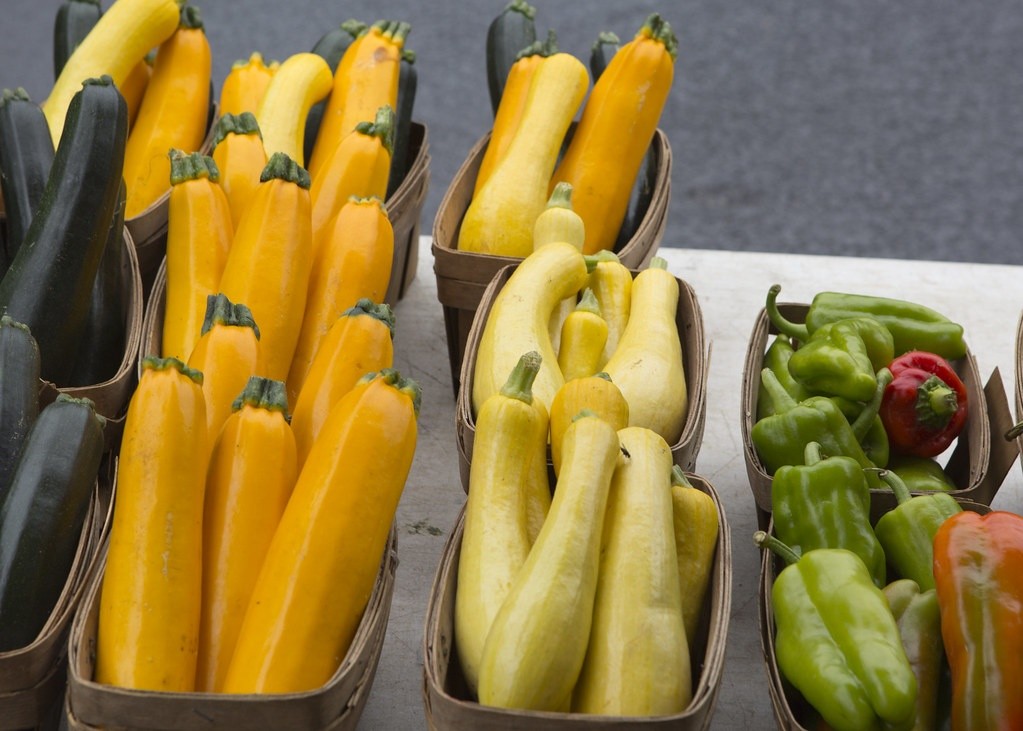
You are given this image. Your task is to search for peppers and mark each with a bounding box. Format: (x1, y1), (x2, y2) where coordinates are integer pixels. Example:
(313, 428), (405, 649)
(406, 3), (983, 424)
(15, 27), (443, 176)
(752, 284), (1023, 731)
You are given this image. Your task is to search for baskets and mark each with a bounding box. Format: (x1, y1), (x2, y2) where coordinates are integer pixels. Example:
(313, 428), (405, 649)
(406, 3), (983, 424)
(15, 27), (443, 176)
(0, 99), (1023, 731)
